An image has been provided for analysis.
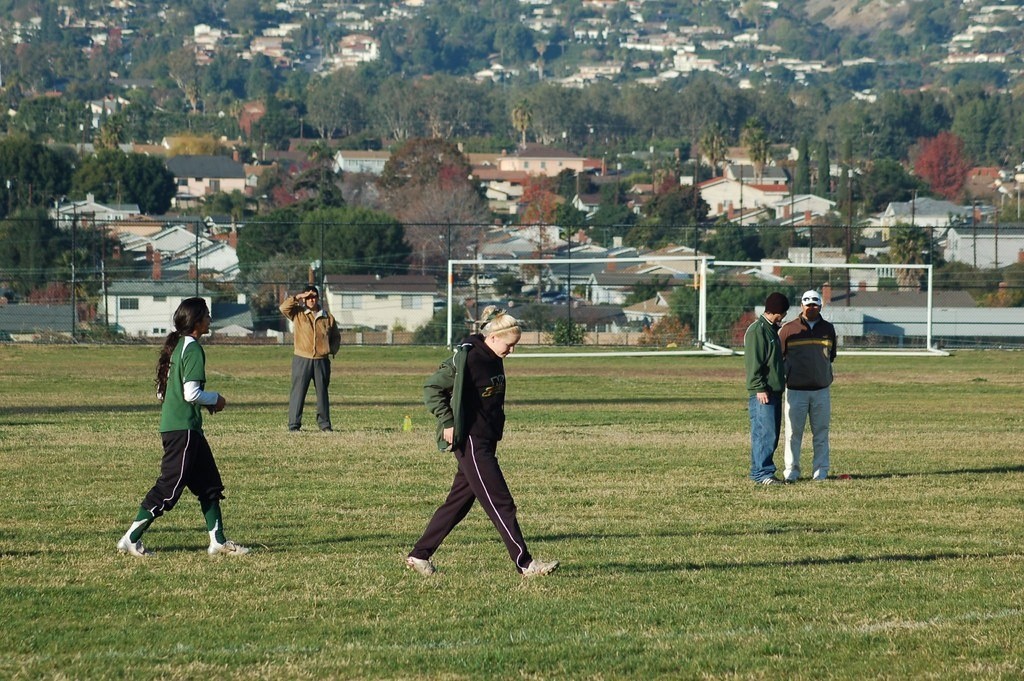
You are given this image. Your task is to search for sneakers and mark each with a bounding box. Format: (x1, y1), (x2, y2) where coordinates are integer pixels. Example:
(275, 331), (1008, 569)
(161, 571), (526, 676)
(522, 559), (560, 578)
(117, 536), (159, 560)
(755, 476), (783, 486)
(405, 557), (436, 576)
(207, 541), (249, 556)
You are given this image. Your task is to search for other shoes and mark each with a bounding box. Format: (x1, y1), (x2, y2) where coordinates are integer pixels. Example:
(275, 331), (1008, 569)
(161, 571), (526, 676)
(321, 427), (333, 433)
(289, 428), (299, 431)
(784, 479), (792, 485)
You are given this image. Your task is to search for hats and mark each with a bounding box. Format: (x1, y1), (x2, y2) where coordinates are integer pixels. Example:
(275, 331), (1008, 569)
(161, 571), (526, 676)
(766, 292), (789, 313)
(801, 290), (822, 306)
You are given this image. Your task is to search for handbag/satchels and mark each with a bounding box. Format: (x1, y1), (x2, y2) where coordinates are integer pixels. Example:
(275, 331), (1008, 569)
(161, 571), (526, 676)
(328, 320), (341, 359)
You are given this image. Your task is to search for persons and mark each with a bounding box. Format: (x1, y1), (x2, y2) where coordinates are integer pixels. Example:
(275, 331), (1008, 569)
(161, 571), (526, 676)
(410, 306), (560, 578)
(117, 298), (250, 557)
(279, 286), (340, 431)
(744, 289), (837, 485)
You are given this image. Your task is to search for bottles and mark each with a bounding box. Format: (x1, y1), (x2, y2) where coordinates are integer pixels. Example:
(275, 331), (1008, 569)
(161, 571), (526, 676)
(402, 416), (412, 431)
